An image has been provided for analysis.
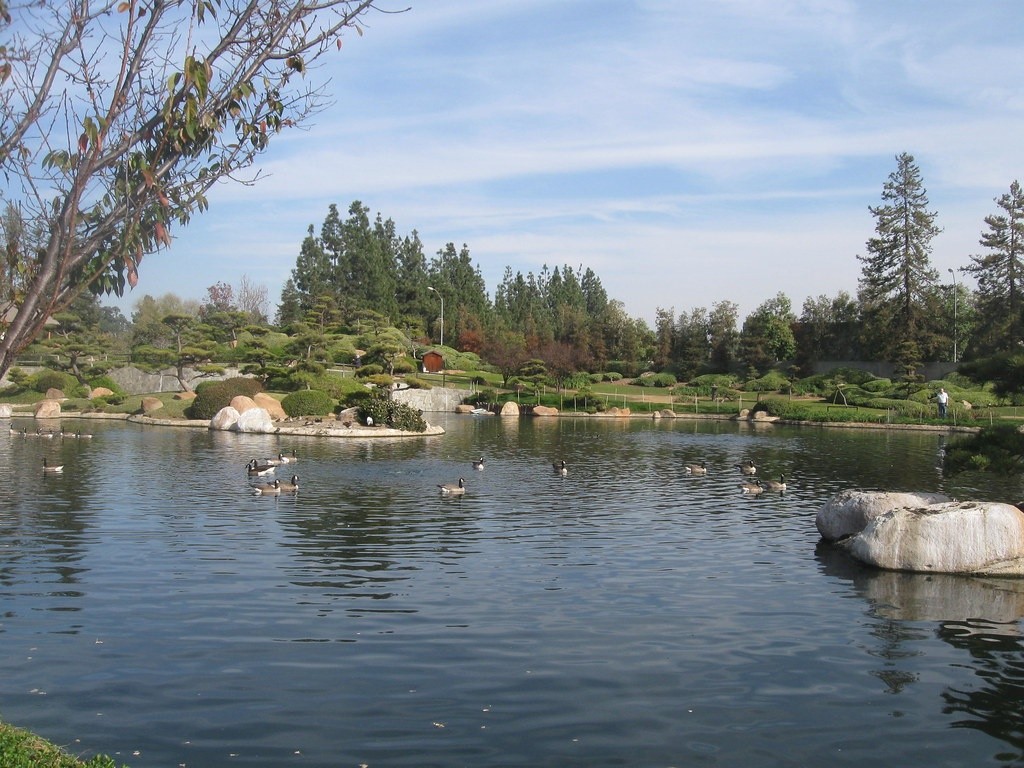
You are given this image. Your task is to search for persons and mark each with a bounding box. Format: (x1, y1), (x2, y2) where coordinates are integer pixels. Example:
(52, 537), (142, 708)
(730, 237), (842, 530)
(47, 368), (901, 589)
(352, 354), (361, 369)
(936, 388), (948, 420)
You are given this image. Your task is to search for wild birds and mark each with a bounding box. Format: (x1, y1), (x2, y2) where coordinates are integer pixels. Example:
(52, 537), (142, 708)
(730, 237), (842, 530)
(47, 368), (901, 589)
(274, 411), (353, 436)
(8, 422), (93, 439)
(245, 459), (276, 477)
(264, 449), (298, 466)
(41, 457), (65, 475)
(685, 460), (707, 478)
(436, 477), (466, 500)
(249, 475), (300, 498)
(472, 457), (484, 472)
(737, 460), (757, 475)
(741, 473), (787, 499)
(552, 460), (568, 478)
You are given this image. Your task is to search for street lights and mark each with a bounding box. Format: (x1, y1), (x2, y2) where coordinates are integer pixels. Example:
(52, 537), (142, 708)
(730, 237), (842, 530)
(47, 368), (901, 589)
(948, 268), (957, 363)
(428, 286), (444, 346)
